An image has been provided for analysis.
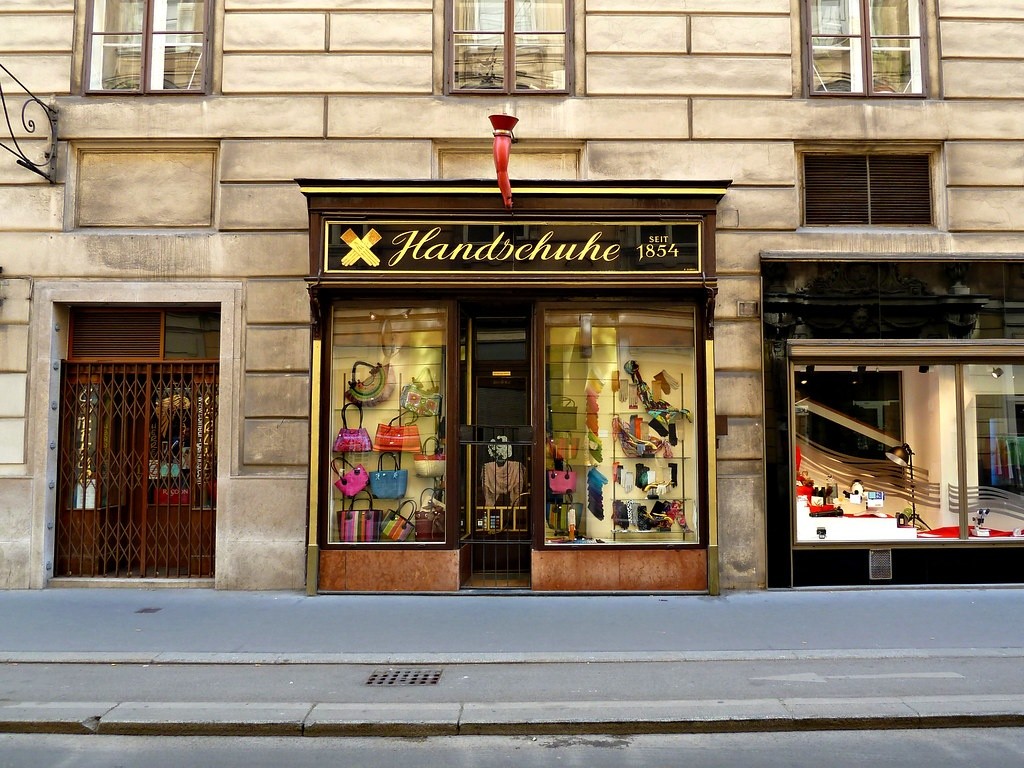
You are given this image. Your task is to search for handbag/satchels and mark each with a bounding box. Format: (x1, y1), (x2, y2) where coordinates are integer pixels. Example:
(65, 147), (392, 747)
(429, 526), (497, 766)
(373, 411), (423, 454)
(336, 489), (383, 542)
(549, 398), (578, 432)
(548, 460), (577, 493)
(369, 452), (408, 499)
(400, 368), (443, 416)
(381, 500), (417, 541)
(414, 488), (445, 542)
(344, 361), (396, 406)
(547, 493), (583, 531)
(332, 403), (373, 451)
(331, 457), (370, 499)
(414, 436), (445, 477)
(551, 429), (579, 458)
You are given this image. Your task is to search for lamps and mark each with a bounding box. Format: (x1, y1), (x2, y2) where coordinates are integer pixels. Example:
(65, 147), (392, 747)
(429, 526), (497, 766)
(369, 311), (377, 320)
(885, 442), (933, 531)
(991, 366), (1004, 380)
(401, 309), (412, 320)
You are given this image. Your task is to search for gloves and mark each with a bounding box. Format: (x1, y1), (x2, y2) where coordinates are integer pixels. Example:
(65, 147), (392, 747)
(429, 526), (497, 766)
(629, 408), (692, 456)
(613, 462), (678, 495)
(613, 501), (685, 531)
(586, 370), (604, 463)
(611, 369), (679, 406)
(587, 466), (608, 521)
